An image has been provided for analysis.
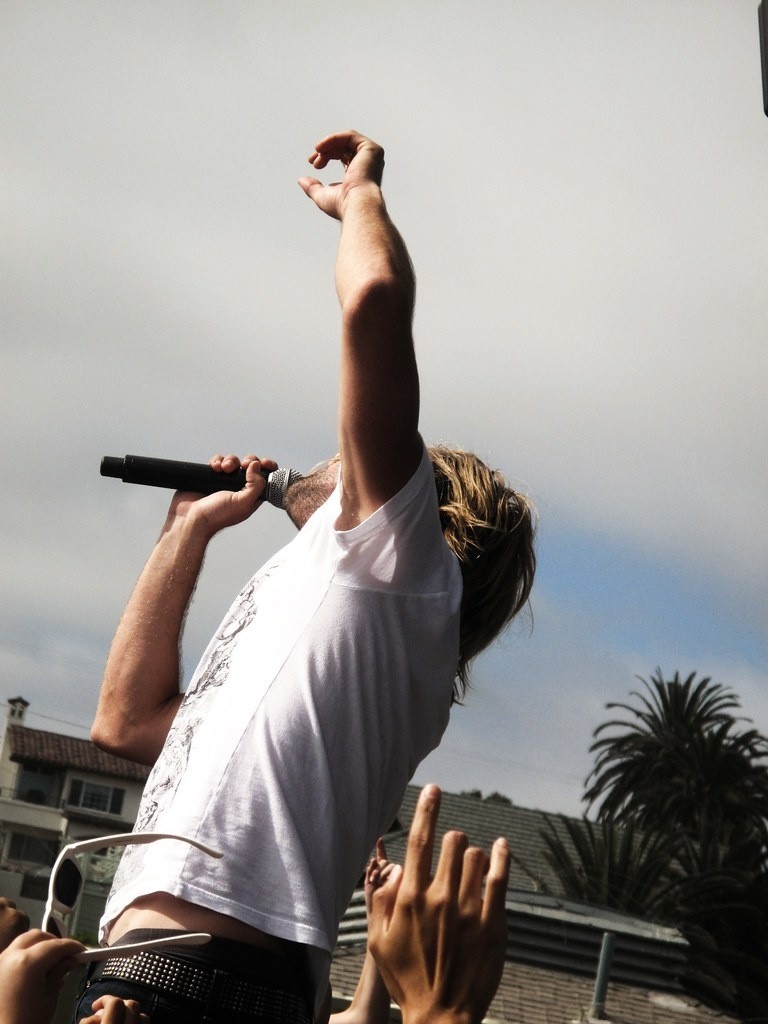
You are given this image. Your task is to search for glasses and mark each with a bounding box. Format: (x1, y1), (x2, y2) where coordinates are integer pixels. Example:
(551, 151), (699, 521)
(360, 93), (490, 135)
(40, 832), (224, 963)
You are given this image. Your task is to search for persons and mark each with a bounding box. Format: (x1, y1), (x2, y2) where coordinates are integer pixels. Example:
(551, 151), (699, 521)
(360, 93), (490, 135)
(82, 132), (537, 1024)
(0, 779), (511, 1024)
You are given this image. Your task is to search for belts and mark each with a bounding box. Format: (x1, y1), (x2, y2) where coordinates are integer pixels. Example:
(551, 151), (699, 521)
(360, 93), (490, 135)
(87, 948), (326, 1024)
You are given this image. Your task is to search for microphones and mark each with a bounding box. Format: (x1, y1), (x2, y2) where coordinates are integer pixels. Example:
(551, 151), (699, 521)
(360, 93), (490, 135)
(98, 454), (302, 509)
